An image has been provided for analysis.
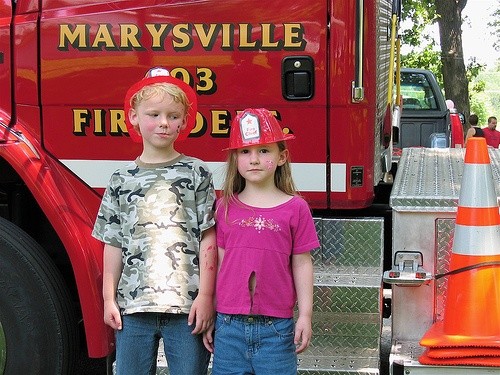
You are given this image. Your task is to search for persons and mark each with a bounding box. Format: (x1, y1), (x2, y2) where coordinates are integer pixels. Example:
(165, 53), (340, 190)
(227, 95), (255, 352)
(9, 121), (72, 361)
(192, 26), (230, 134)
(482, 116), (500, 149)
(92, 67), (217, 375)
(201, 108), (320, 375)
(465, 114), (483, 148)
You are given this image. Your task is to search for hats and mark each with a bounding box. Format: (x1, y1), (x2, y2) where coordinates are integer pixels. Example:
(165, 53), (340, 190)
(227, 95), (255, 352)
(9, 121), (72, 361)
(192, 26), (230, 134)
(125, 68), (196, 143)
(221, 108), (295, 151)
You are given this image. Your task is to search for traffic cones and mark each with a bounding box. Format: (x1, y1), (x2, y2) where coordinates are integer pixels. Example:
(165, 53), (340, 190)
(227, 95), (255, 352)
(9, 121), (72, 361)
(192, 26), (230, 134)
(418, 137), (500, 366)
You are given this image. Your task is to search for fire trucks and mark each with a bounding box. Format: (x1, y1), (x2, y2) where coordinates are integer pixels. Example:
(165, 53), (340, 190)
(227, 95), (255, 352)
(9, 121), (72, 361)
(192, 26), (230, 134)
(0, 0), (500, 375)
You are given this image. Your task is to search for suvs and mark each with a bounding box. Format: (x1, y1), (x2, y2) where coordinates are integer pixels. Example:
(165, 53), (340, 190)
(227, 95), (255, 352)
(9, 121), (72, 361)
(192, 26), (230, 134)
(392, 67), (451, 155)
